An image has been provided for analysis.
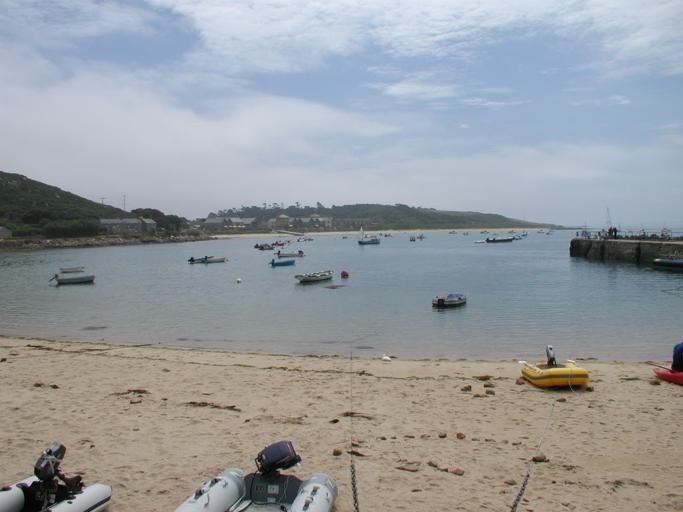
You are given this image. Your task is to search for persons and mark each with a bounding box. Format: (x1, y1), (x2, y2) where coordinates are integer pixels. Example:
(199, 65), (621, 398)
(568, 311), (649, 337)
(670, 342), (682, 373)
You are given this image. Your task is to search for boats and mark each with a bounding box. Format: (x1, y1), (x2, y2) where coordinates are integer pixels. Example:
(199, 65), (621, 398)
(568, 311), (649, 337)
(47, 266), (97, 286)
(293, 270), (334, 284)
(537, 228), (555, 236)
(652, 257), (683, 268)
(447, 227), (470, 236)
(474, 228), (529, 244)
(253, 227), (314, 267)
(187, 255), (227, 264)
(432, 292), (466, 307)
(650, 366), (683, 385)
(340, 226), (393, 245)
(519, 359), (593, 389)
(174, 440), (340, 512)
(0, 440), (115, 512)
(409, 231), (425, 242)
